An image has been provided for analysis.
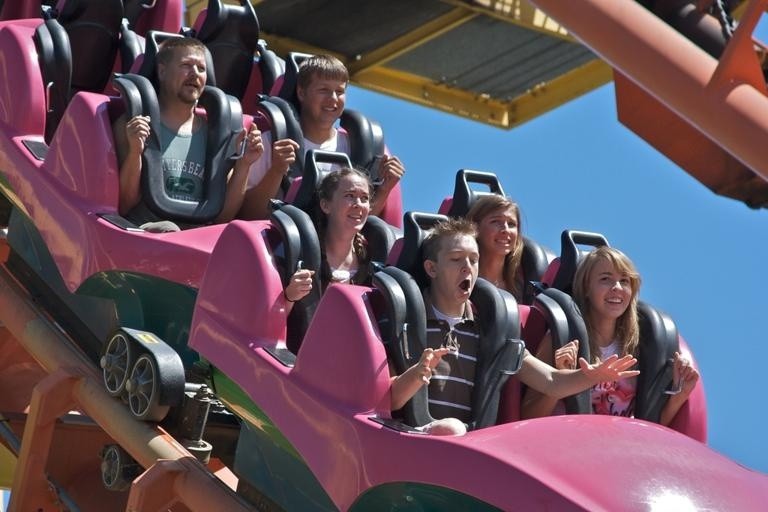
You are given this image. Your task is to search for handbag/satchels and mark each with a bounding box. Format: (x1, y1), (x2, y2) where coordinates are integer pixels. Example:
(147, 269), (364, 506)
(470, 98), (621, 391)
(283, 289), (299, 303)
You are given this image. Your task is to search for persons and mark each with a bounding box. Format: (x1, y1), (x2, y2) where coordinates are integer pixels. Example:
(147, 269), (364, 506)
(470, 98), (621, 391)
(109, 35), (265, 235)
(377, 216), (642, 432)
(464, 192), (525, 306)
(519, 246), (701, 427)
(265, 168), (377, 359)
(238, 53), (407, 220)
